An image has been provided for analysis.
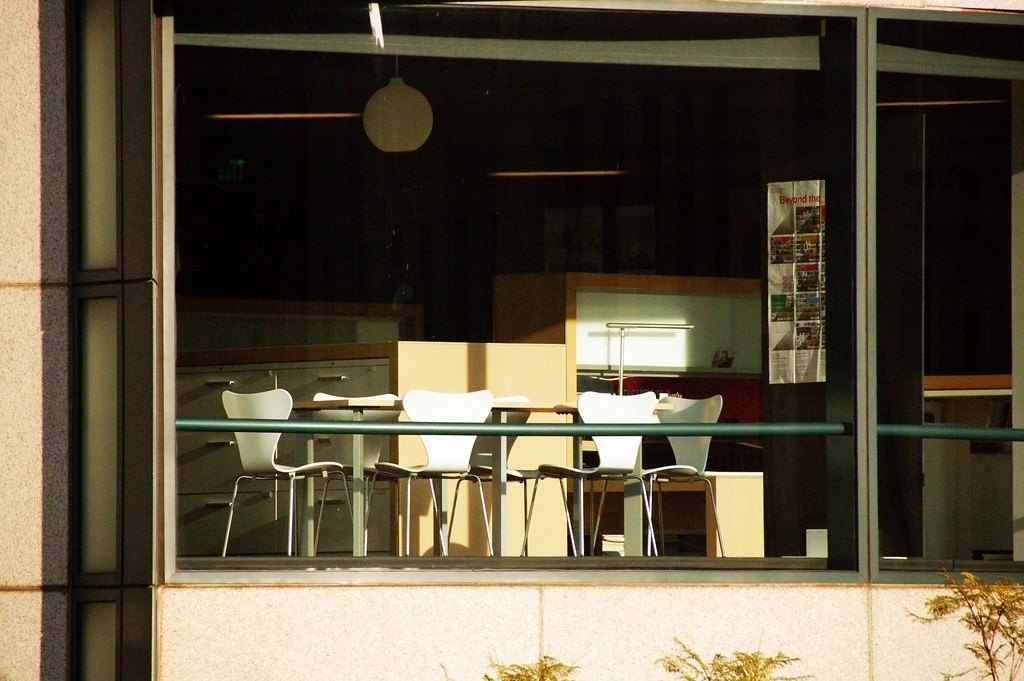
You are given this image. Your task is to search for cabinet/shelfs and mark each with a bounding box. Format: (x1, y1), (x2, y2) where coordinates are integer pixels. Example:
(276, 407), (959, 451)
(563, 470), (768, 559)
(177, 340), (574, 558)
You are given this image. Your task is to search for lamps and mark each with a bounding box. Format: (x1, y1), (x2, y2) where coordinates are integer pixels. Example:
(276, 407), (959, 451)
(360, 56), (434, 153)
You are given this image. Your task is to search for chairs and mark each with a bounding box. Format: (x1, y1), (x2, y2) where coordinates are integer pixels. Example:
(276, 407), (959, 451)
(589, 394), (727, 558)
(441, 395), (534, 557)
(219, 388), (352, 558)
(363, 388), (497, 559)
(519, 390), (660, 557)
(310, 391), (406, 558)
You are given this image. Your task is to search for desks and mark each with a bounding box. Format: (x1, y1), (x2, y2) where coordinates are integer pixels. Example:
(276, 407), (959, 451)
(289, 396), (676, 557)
